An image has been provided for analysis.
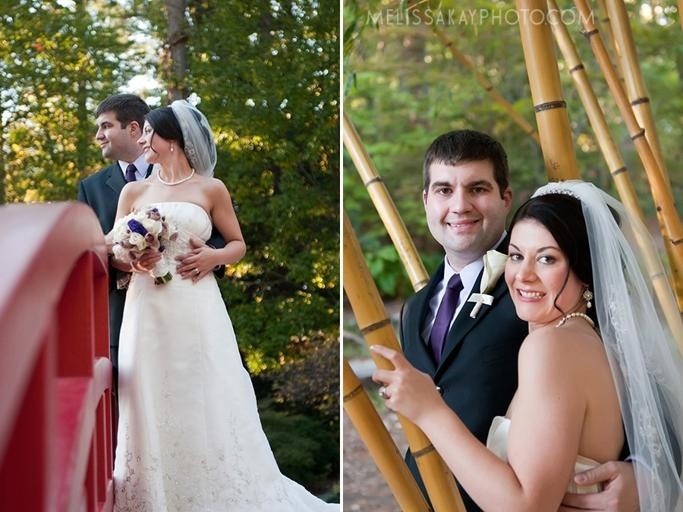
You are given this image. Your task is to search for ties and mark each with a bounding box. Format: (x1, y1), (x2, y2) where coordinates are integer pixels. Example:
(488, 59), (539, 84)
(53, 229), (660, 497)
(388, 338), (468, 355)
(424, 273), (463, 367)
(124, 164), (138, 183)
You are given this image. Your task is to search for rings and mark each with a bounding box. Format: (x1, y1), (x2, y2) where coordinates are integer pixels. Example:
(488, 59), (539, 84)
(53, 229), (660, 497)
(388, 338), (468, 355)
(377, 385), (390, 399)
(195, 267), (200, 274)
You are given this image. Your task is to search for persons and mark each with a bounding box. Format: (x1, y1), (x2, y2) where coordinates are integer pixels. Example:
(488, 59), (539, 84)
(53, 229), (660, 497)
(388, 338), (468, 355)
(370, 180), (683, 511)
(75, 93), (225, 376)
(113, 91), (341, 511)
(399, 128), (683, 511)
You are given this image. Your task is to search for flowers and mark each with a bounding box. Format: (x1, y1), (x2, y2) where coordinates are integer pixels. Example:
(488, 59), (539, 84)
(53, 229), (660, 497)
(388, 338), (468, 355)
(469, 250), (509, 318)
(112, 207), (180, 287)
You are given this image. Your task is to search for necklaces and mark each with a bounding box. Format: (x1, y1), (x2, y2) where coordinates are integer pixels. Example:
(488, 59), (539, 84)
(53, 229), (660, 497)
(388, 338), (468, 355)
(157, 168), (195, 185)
(556, 311), (595, 330)
